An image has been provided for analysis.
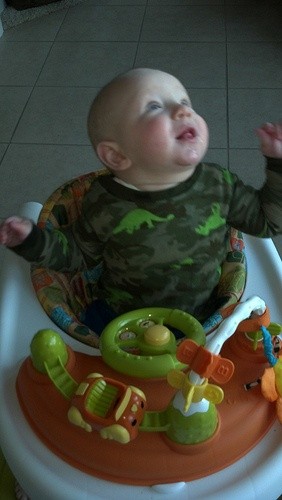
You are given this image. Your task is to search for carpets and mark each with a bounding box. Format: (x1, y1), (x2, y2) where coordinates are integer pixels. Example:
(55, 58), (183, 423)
(0, 0), (85, 30)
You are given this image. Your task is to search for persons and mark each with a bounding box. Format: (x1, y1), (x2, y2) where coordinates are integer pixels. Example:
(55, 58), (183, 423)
(0, 68), (282, 341)
